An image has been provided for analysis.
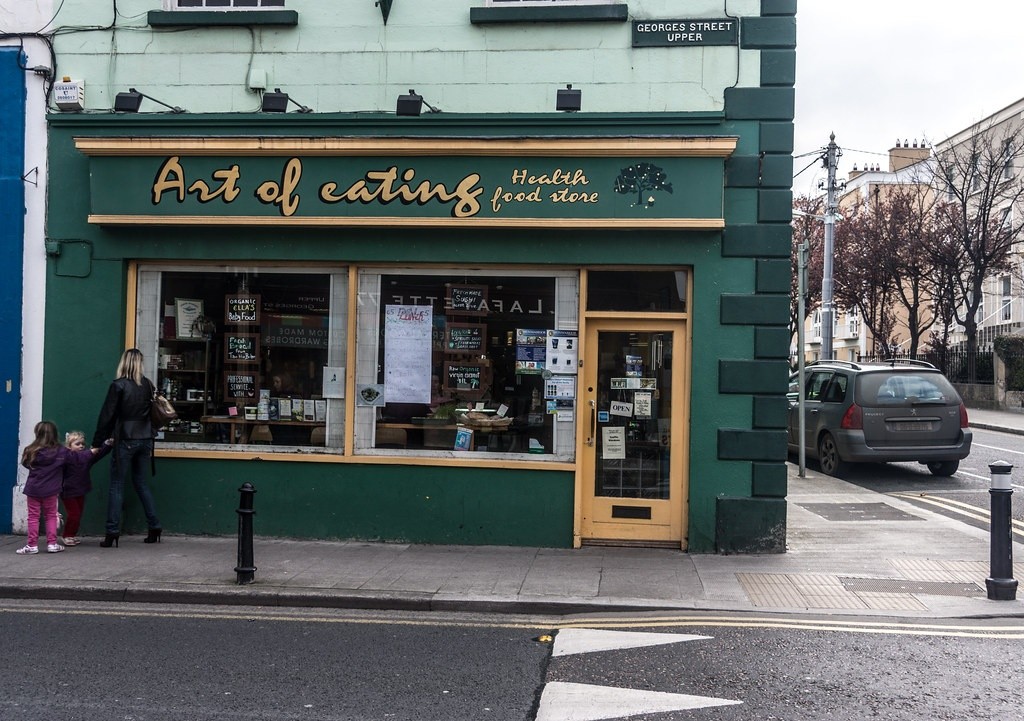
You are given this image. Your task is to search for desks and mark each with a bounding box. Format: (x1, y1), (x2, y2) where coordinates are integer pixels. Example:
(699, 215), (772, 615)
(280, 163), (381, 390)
(202, 417), (326, 445)
(376, 422), (509, 453)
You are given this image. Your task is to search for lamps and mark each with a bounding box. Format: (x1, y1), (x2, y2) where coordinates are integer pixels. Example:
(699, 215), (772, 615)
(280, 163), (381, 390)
(556, 84), (581, 110)
(261, 88), (311, 114)
(397, 88), (435, 117)
(113, 88), (183, 115)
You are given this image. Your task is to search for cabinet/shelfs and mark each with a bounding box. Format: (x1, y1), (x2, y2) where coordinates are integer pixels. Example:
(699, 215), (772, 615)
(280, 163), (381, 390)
(159, 337), (220, 443)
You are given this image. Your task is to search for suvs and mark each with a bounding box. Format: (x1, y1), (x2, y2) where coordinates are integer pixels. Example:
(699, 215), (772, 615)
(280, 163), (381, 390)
(785, 359), (973, 476)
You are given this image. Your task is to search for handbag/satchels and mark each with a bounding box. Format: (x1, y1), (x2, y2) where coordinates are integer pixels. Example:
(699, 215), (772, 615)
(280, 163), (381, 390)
(147, 378), (176, 430)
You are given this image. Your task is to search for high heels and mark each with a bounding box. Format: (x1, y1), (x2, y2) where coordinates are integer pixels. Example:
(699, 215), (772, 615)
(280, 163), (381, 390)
(99, 531), (119, 548)
(144, 527), (163, 543)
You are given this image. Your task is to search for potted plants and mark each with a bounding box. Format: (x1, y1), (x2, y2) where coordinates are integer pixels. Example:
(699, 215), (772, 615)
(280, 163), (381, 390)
(434, 404), (456, 419)
(190, 312), (216, 338)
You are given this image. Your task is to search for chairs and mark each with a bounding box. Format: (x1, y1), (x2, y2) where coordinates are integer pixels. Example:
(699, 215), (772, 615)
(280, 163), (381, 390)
(820, 380), (843, 401)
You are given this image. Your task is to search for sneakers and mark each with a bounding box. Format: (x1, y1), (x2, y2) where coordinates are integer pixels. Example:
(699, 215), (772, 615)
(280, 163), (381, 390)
(62, 536), (75, 546)
(16, 544), (38, 554)
(47, 543), (65, 553)
(72, 536), (81, 545)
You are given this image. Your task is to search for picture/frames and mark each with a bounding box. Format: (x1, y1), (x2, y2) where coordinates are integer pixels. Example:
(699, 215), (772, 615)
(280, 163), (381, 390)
(224, 295), (261, 326)
(447, 283), (489, 316)
(444, 322), (486, 354)
(223, 371), (260, 403)
(174, 298), (205, 341)
(443, 360), (484, 393)
(223, 333), (261, 365)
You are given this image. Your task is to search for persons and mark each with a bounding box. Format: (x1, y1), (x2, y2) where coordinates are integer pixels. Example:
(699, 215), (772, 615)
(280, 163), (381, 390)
(89, 349), (163, 548)
(58, 431), (114, 546)
(425, 374), (455, 414)
(270, 371), (304, 445)
(15, 421), (100, 554)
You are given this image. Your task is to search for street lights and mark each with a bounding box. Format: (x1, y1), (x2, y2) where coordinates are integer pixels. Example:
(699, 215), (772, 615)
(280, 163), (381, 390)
(792, 209), (833, 387)
